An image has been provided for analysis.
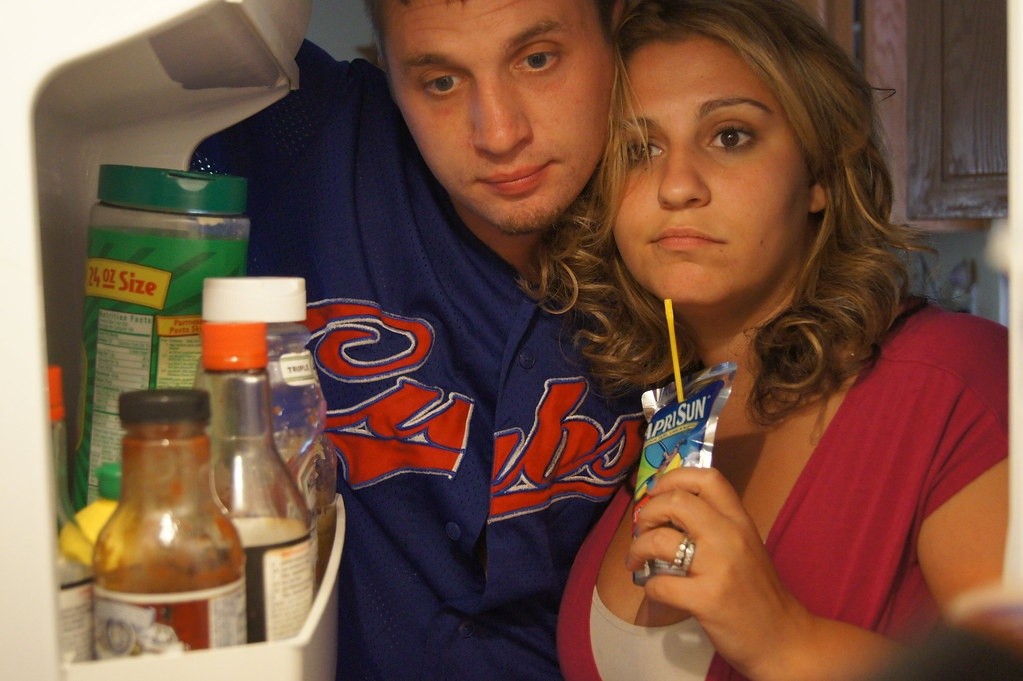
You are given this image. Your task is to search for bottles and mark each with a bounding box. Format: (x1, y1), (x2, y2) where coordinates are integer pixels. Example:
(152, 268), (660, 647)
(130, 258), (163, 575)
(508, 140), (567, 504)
(74, 163), (251, 510)
(49, 365), (94, 662)
(194, 277), (338, 590)
(198, 320), (316, 647)
(62, 462), (125, 568)
(91, 389), (248, 659)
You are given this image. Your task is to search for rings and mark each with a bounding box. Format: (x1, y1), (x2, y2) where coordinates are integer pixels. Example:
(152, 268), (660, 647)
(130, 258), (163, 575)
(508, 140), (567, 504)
(670, 536), (696, 576)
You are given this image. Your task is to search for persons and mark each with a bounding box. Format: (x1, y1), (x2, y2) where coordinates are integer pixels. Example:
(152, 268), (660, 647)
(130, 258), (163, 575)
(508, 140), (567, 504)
(187, 0), (706, 681)
(517, 0), (1023, 681)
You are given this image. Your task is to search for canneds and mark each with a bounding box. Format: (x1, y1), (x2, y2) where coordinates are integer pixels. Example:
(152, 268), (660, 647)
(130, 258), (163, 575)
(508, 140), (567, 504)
(73, 162), (252, 512)
